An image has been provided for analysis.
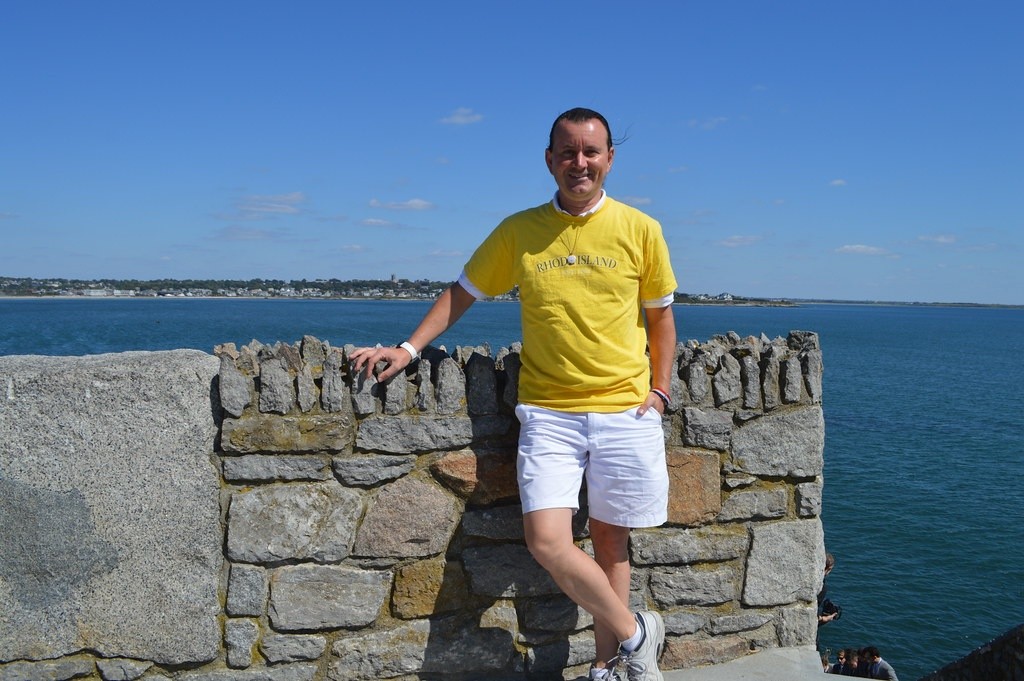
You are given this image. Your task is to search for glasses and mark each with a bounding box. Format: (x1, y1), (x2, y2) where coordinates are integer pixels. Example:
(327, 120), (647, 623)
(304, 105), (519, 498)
(837, 657), (844, 660)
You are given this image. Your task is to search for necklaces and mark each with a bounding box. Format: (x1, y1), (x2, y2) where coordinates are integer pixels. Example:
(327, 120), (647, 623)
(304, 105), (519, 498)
(561, 210), (587, 265)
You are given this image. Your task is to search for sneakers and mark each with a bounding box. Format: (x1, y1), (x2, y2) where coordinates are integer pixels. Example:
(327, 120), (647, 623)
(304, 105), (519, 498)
(590, 611), (668, 680)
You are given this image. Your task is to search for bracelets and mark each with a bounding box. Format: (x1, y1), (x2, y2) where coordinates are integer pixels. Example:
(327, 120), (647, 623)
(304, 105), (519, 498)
(396, 342), (418, 363)
(650, 388), (670, 406)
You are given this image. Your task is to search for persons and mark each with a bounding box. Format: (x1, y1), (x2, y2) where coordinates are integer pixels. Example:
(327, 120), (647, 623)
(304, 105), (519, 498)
(816, 553), (837, 651)
(821, 647), (898, 681)
(349, 108), (676, 680)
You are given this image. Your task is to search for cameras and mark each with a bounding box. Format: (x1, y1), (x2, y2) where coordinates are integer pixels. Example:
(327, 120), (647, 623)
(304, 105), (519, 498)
(822, 599), (841, 620)
